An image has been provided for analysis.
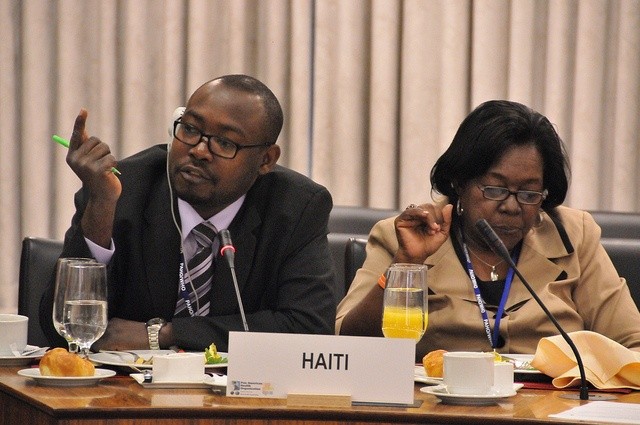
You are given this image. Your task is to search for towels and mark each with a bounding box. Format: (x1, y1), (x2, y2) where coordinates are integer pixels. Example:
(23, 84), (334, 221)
(528, 329), (640, 392)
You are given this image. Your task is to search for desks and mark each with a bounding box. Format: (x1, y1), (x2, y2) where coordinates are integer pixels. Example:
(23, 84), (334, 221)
(0, 359), (640, 420)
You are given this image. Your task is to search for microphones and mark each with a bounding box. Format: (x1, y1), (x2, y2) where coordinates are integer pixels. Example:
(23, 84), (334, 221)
(218, 228), (252, 332)
(475, 218), (591, 399)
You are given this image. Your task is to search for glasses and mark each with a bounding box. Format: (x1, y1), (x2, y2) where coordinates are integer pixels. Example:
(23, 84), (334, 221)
(173, 116), (273, 159)
(475, 179), (548, 205)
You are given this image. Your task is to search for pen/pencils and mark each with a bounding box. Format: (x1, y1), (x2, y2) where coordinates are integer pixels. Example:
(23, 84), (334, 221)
(51, 135), (122, 175)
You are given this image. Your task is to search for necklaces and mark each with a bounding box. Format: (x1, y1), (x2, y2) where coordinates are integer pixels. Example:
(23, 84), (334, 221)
(465, 243), (507, 281)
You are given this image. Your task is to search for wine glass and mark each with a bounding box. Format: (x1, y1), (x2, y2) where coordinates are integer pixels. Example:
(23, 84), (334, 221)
(63, 262), (109, 361)
(390, 263), (429, 335)
(52, 257), (96, 353)
(381, 267), (426, 345)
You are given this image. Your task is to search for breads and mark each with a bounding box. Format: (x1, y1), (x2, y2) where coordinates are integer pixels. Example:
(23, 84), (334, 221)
(39, 347), (96, 378)
(422, 350), (449, 377)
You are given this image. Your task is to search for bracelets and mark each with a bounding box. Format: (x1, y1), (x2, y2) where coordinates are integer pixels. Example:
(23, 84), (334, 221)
(376, 273), (387, 291)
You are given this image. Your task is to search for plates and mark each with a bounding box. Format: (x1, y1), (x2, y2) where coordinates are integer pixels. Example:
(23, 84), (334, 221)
(419, 385), (517, 406)
(0, 345), (47, 367)
(499, 353), (544, 374)
(163, 352), (228, 373)
(129, 373), (227, 389)
(414, 367), (443, 385)
(17, 368), (116, 386)
(88, 350), (176, 372)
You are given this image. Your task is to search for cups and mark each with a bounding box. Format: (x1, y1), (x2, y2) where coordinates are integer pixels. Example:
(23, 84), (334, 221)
(0, 314), (29, 356)
(442, 352), (494, 396)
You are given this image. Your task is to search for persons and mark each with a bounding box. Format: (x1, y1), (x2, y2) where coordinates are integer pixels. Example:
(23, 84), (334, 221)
(37, 74), (330, 352)
(335, 100), (639, 356)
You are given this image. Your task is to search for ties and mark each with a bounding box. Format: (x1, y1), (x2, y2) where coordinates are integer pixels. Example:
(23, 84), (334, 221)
(175, 223), (220, 318)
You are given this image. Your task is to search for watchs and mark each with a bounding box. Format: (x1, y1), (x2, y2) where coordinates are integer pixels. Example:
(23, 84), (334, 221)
(144, 317), (167, 350)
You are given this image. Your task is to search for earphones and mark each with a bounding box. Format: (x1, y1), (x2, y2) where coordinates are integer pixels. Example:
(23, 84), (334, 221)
(166, 107), (185, 138)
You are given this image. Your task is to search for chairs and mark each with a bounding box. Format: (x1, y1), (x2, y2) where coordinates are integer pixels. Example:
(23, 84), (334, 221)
(343, 237), (369, 298)
(19, 237), (68, 352)
